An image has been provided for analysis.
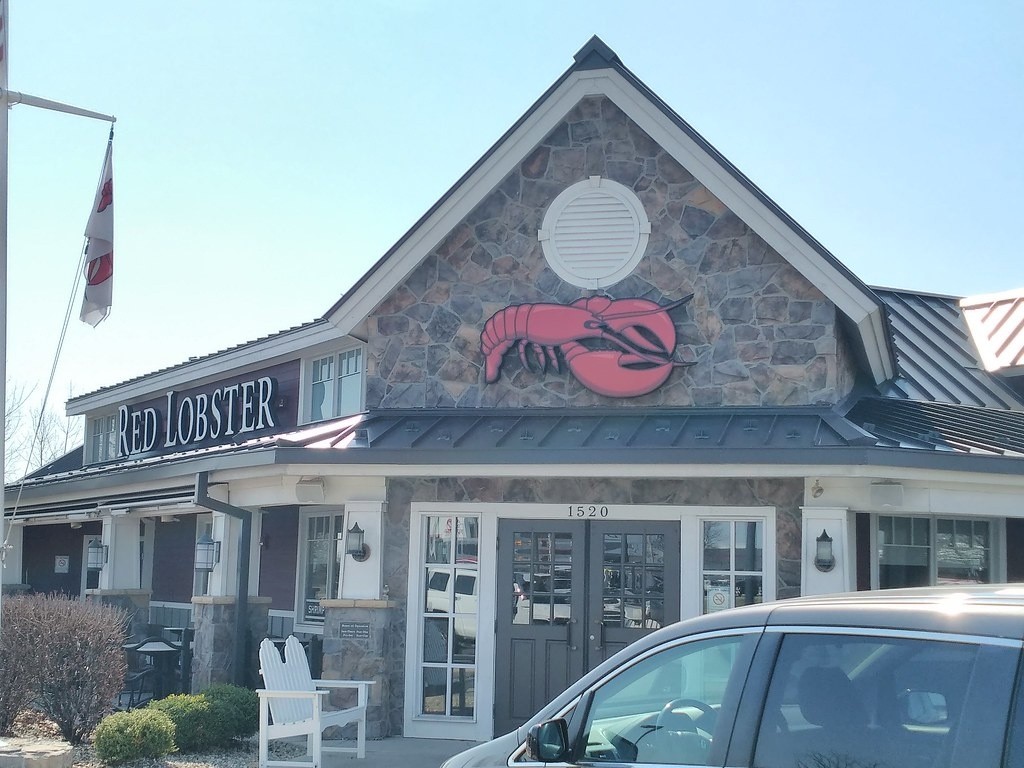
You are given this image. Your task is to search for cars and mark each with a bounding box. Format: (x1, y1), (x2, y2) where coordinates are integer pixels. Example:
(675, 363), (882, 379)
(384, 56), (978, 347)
(705, 579), (742, 597)
(553, 579), (570, 593)
(435, 583), (1024, 768)
(426, 564), (622, 658)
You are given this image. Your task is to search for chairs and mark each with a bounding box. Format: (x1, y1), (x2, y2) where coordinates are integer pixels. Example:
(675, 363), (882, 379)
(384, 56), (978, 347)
(304, 633), (322, 679)
(117, 634), (194, 713)
(799, 664), (871, 729)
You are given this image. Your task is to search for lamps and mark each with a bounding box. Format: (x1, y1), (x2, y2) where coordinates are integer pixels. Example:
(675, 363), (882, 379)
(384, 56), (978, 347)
(193, 528), (221, 572)
(811, 479), (824, 498)
(814, 528), (836, 572)
(87, 538), (109, 570)
(345, 522), (372, 563)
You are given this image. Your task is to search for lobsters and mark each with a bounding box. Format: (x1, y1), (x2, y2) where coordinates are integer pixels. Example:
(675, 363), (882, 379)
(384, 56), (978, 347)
(480, 289), (696, 400)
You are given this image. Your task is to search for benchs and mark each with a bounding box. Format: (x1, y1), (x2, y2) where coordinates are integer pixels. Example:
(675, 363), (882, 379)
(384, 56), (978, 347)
(254, 634), (378, 768)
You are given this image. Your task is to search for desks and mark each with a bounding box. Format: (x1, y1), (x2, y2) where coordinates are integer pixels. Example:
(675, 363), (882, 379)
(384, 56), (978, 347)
(123, 642), (195, 699)
(270, 637), (310, 663)
(164, 626), (195, 641)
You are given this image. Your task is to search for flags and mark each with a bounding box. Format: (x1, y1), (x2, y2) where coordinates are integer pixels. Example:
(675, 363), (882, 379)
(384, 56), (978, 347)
(79, 138), (116, 326)
